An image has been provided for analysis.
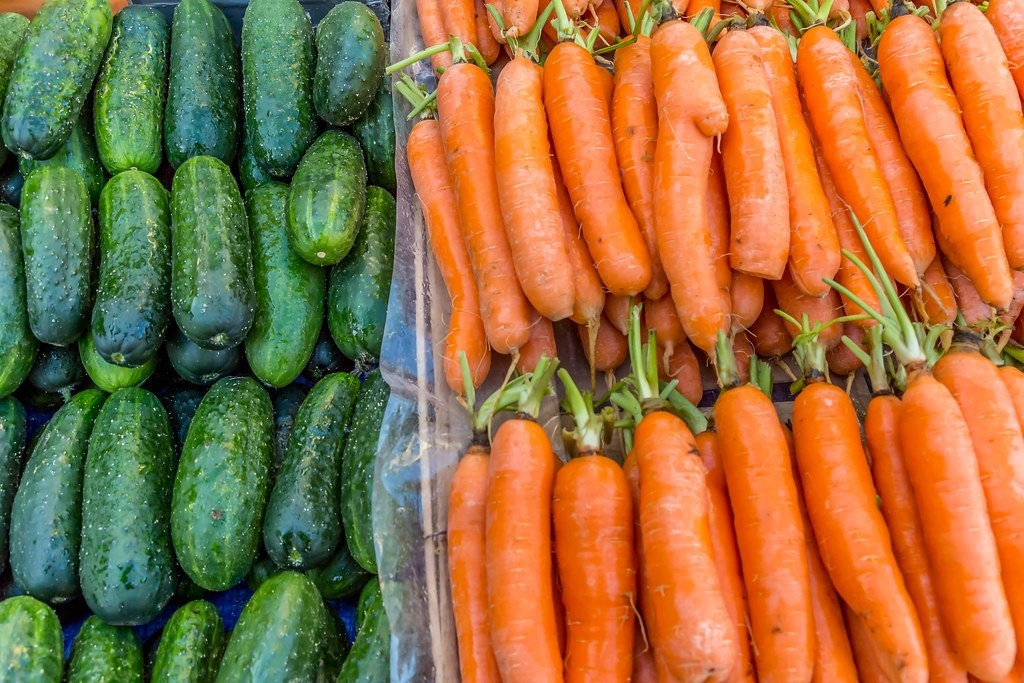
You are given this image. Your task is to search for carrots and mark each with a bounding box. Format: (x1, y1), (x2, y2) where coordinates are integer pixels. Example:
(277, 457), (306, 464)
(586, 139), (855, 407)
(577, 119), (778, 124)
(406, 0), (1024, 683)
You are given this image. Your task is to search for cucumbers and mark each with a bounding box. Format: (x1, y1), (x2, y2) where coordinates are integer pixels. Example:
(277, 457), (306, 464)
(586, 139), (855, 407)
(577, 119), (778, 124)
(1, 0), (430, 683)
